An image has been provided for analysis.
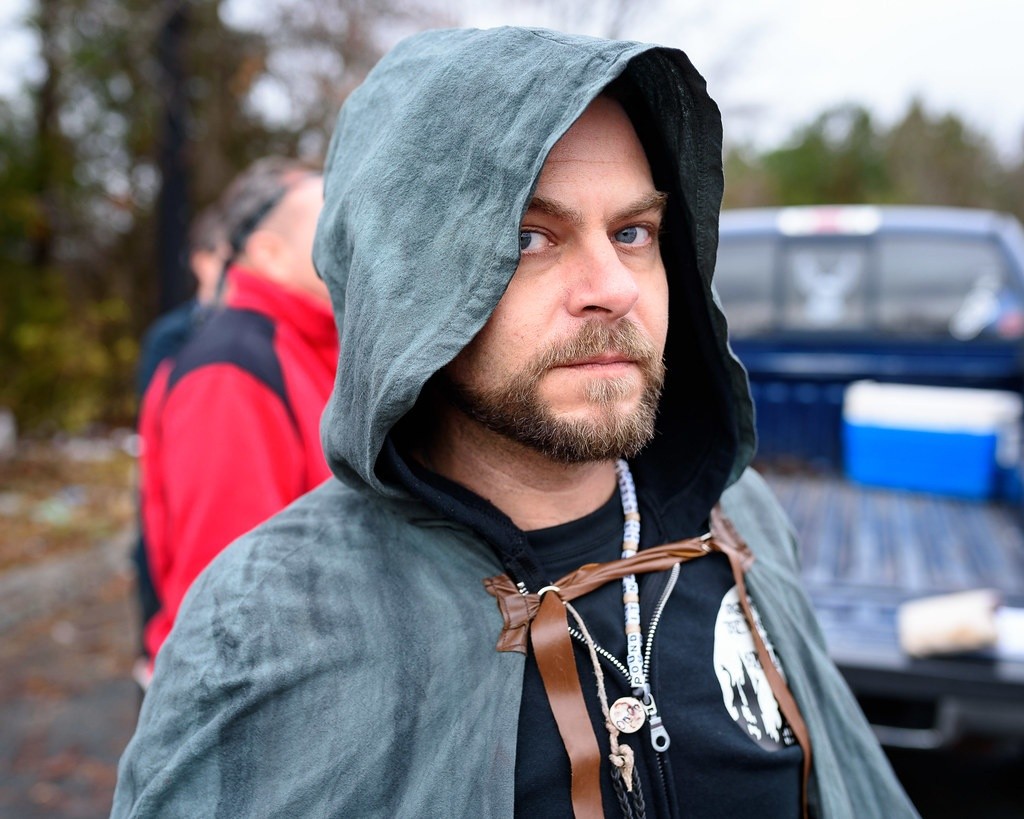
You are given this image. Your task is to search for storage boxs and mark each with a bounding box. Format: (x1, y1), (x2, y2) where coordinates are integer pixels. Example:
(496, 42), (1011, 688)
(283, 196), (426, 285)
(839, 379), (1022, 501)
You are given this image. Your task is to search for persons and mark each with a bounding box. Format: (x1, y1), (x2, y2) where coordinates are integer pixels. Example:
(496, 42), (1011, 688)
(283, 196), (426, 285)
(130, 156), (337, 699)
(111, 26), (923, 819)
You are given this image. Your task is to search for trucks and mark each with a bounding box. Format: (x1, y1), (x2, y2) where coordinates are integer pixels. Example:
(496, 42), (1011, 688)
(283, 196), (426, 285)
(709, 204), (1024, 783)
